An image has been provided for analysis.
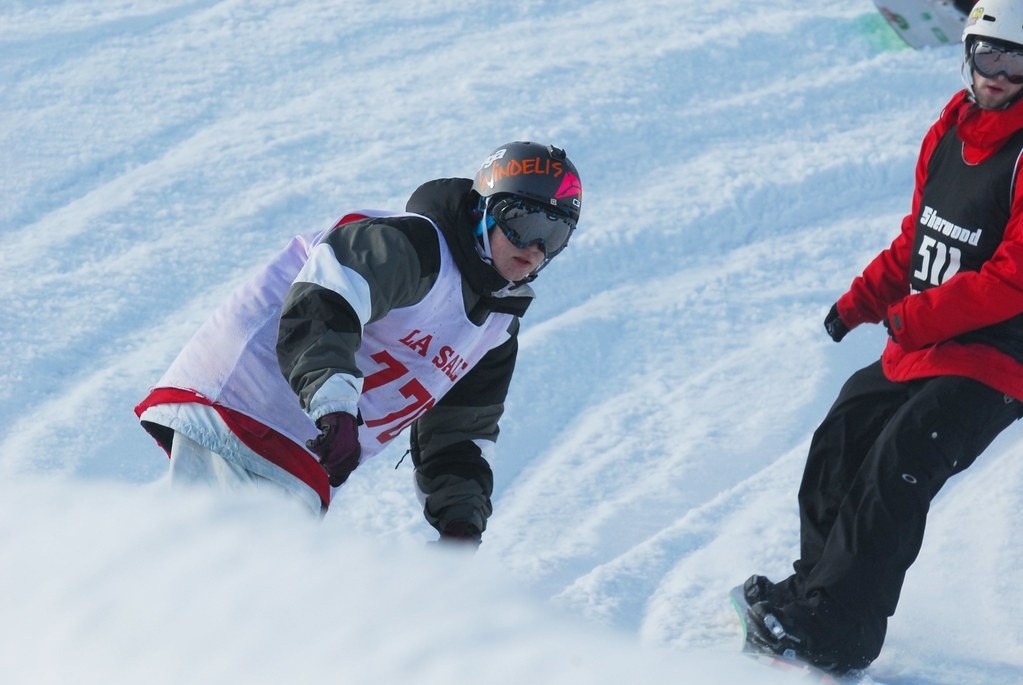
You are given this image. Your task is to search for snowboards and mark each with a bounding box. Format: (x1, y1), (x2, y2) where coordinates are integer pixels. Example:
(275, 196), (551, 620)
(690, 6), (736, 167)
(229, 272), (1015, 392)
(726, 578), (862, 685)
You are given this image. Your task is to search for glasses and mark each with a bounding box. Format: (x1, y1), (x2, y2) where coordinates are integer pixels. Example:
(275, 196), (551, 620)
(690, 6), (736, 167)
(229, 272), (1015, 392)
(490, 198), (575, 258)
(969, 40), (1023, 84)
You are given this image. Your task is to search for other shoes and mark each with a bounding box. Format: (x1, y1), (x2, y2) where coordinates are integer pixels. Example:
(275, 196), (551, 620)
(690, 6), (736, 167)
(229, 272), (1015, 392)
(746, 575), (856, 677)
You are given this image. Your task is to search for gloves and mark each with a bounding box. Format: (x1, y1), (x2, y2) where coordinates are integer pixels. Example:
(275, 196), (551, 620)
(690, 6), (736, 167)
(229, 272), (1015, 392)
(883, 318), (898, 344)
(824, 302), (852, 344)
(306, 412), (362, 489)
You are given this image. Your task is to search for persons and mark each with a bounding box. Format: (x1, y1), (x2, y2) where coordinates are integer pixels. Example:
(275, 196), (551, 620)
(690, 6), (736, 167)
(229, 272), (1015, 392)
(134, 140), (580, 555)
(731, 0), (1023, 674)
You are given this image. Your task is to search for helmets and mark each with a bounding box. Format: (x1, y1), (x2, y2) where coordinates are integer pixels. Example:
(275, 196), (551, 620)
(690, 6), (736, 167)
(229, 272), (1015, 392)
(467, 141), (584, 219)
(962, 0), (1023, 48)
